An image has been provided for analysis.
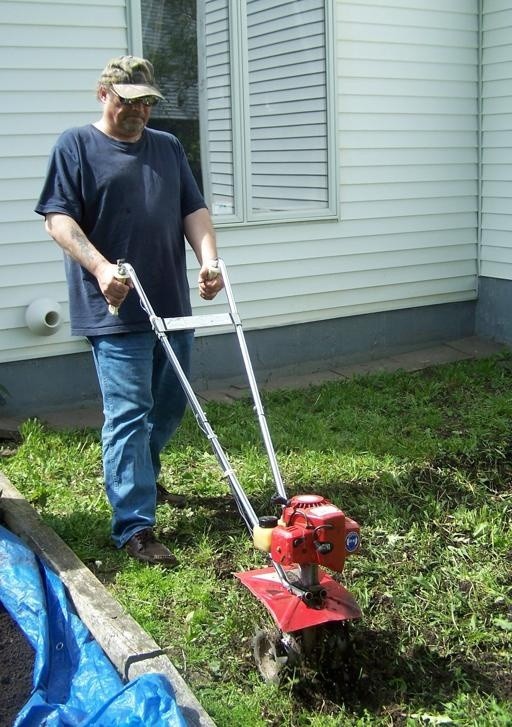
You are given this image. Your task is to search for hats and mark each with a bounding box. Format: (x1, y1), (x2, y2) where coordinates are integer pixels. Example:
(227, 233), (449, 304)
(97, 55), (166, 100)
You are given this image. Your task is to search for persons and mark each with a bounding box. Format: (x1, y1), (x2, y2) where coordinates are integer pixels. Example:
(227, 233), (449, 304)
(29, 52), (224, 569)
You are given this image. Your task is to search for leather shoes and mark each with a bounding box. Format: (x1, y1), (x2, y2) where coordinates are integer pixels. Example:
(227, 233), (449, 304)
(125, 529), (178, 568)
(157, 481), (187, 508)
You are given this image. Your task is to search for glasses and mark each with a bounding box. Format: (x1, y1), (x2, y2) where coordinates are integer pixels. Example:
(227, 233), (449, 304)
(110, 89), (160, 106)
